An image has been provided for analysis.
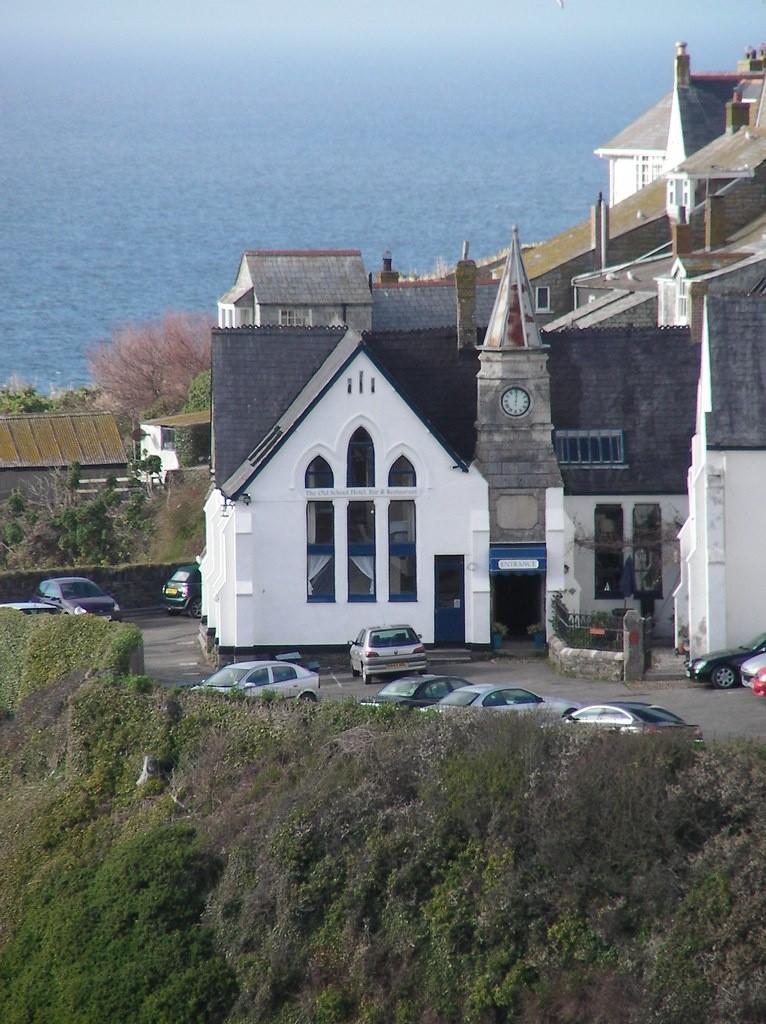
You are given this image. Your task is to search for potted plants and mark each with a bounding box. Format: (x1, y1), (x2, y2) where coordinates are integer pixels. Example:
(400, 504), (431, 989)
(492, 621), (508, 649)
(529, 622), (546, 648)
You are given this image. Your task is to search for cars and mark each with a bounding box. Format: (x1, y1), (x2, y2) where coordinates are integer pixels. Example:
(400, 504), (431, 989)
(29, 577), (122, 624)
(191, 661), (324, 702)
(751, 666), (766, 697)
(685, 633), (766, 688)
(740, 653), (766, 686)
(562, 702), (704, 750)
(418, 683), (582, 720)
(1, 602), (62, 617)
(162, 565), (203, 619)
(359, 675), (474, 712)
(347, 623), (428, 683)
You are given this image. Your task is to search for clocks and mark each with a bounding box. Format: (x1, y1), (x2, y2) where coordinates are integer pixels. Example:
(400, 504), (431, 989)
(498, 384), (534, 419)
